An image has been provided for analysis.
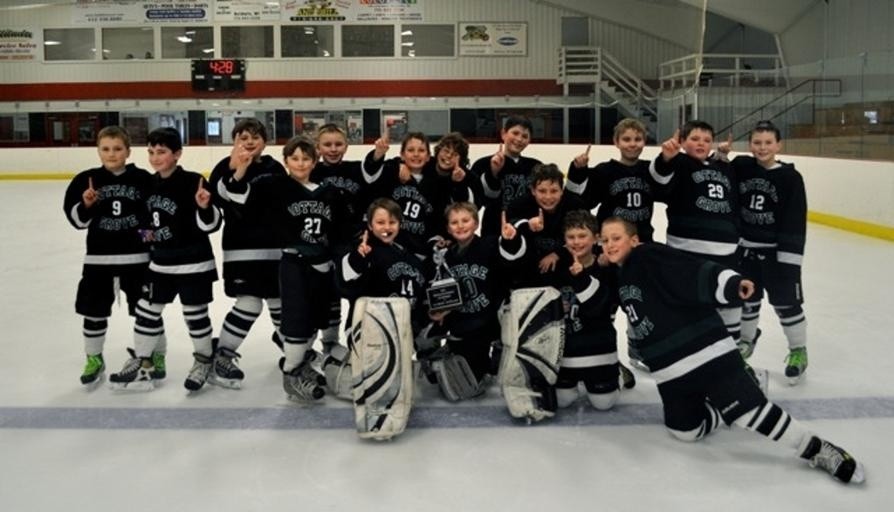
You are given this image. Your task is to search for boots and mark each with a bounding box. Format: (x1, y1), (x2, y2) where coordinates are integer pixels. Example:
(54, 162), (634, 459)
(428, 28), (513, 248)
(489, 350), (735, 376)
(735, 328), (762, 359)
(273, 332), (351, 402)
(784, 346), (808, 377)
(618, 361), (635, 389)
(800, 437), (856, 483)
(185, 338), (244, 391)
(81, 353), (105, 384)
(109, 348), (166, 384)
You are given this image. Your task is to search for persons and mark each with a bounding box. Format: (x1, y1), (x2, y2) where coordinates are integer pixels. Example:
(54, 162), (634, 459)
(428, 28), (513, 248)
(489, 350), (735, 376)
(647, 121), (759, 388)
(110, 127), (221, 391)
(316, 111), (634, 442)
(564, 117), (671, 364)
(208, 118), (319, 383)
(593, 216), (864, 486)
(64, 126), (166, 385)
(713, 119), (810, 378)
(226, 137), (349, 403)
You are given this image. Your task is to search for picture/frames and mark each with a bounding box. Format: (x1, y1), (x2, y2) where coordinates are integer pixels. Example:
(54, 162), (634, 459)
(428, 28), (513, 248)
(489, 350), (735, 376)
(457, 20), (529, 58)
